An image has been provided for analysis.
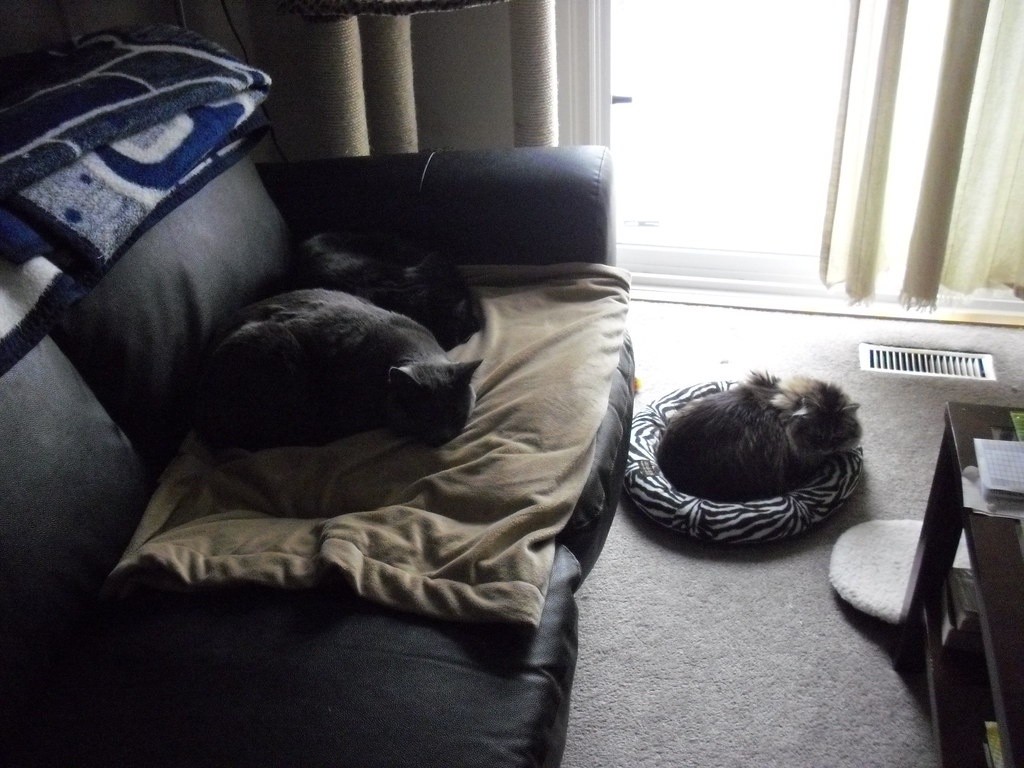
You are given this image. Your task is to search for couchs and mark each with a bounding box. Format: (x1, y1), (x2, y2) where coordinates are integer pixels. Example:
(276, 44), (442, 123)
(0, 111), (638, 768)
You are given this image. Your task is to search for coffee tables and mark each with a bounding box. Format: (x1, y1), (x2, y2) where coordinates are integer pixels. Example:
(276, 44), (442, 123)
(893, 401), (1024, 768)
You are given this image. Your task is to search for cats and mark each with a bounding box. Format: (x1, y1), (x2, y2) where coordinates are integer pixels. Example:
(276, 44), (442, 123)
(654, 369), (863, 504)
(210, 288), (483, 450)
